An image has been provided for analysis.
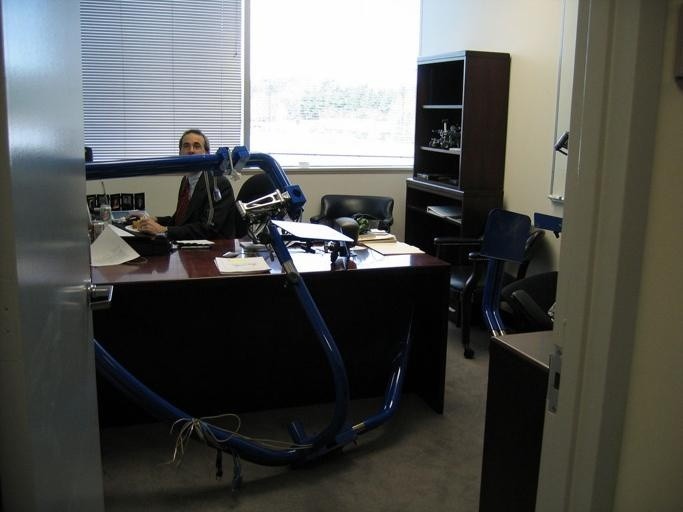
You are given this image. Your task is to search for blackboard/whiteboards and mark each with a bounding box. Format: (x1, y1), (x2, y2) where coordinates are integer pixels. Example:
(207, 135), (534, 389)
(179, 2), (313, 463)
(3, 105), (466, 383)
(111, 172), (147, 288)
(548, 0), (581, 204)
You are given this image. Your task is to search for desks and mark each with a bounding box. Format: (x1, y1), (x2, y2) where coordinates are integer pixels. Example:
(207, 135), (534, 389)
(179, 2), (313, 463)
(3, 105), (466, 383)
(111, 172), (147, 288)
(91, 239), (448, 428)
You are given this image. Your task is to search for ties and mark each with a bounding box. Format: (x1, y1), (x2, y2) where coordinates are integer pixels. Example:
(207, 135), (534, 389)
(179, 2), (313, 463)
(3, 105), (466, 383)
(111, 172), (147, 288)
(173, 178), (191, 225)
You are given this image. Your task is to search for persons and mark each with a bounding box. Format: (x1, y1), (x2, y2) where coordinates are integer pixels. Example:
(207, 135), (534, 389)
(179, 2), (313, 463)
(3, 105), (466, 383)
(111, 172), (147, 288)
(123, 130), (245, 238)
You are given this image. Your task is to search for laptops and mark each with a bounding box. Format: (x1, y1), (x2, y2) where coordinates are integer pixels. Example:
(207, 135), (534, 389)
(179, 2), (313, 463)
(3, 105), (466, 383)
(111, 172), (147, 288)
(87, 202), (136, 238)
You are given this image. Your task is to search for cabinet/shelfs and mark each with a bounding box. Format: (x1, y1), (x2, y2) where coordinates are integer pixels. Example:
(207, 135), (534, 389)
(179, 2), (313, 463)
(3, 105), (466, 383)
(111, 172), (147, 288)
(404, 50), (511, 325)
(477, 329), (555, 512)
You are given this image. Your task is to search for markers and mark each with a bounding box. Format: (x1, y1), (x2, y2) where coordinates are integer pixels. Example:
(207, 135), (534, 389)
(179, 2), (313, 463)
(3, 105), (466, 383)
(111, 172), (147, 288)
(549, 193), (562, 201)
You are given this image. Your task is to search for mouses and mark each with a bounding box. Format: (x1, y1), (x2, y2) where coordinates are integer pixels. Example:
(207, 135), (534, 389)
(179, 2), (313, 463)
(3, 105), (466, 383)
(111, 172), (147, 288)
(126, 214), (140, 221)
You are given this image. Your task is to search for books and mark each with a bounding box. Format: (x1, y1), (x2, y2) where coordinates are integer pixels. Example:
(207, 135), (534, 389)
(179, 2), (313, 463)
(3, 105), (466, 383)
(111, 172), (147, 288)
(213, 254), (272, 275)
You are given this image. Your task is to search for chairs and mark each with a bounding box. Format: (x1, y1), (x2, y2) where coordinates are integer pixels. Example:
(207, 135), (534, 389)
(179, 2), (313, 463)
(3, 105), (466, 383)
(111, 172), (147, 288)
(235, 174), (395, 243)
(435, 210), (557, 338)
